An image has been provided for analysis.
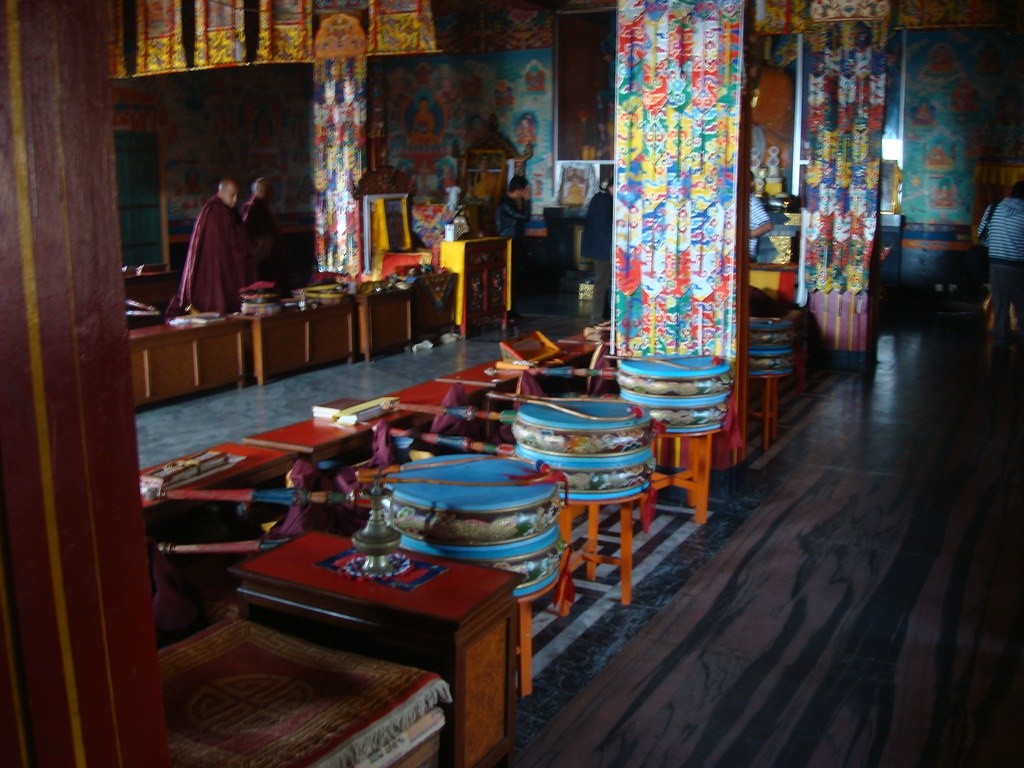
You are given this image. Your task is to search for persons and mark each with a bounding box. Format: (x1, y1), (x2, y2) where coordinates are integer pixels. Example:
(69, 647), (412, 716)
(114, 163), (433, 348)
(747, 169), (774, 267)
(495, 176), (535, 323)
(240, 173), (283, 285)
(974, 179), (1024, 355)
(166, 178), (261, 323)
(578, 176), (613, 327)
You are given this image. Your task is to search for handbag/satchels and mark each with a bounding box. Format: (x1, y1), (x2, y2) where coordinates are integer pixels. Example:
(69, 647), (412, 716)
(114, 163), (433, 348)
(969, 243), (990, 272)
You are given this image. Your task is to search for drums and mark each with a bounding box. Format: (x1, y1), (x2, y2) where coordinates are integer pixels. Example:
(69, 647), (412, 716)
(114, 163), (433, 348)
(748, 315), (796, 377)
(381, 453), (568, 598)
(511, 393), (656, 500)
(615, 354), (734, 434)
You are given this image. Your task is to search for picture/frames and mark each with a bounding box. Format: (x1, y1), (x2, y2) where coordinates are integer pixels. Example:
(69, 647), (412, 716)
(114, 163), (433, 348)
(461, 147), (506, 232)
(377, 197), (412, 251)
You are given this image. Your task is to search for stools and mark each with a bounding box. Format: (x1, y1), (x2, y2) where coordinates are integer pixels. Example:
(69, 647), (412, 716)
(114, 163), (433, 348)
(638, 429), (726, 531)
(553, 489), (647, 616)
(516, 565), (564, 697)
(747, 372), (793, 450)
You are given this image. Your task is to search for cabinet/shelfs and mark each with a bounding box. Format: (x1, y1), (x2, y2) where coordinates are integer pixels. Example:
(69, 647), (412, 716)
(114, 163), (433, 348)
(411, 271), (461, 336)
(123, 270), (182, 306)
(557, 333), (594, 344)
(129, 315), (248, 408)
(434, 344), (599, 438)
(351, 288), (411, 363)
(138, 440), (299, 586)
(243, 378), (490, 488)
(226, 303), (354, 386)
(228, 530), (524, 768)
(440, 236), (512, 339)
